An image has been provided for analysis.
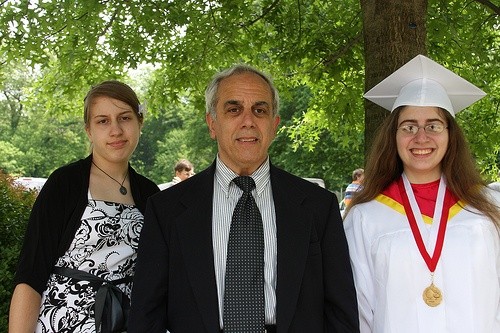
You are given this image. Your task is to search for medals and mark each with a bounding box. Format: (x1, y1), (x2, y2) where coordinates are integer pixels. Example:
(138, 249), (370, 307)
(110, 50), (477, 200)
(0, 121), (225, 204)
(422, 281), (443, 307)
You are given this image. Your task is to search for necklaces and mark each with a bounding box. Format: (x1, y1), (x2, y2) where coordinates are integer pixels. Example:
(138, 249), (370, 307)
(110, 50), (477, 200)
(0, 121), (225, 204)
(91, 161), (127, 195)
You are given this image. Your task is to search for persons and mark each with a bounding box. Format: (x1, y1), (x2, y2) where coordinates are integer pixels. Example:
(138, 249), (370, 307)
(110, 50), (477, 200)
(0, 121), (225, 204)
(8, 81), (161, 333)
(343, 54), (500, 333)
(128, 64), (360, 333)
(157, 160), (194, 190)
(344, 168), (365, 207)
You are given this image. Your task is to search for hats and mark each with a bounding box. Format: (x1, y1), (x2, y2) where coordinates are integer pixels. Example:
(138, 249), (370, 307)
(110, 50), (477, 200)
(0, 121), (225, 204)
(363, 54), (487, 119)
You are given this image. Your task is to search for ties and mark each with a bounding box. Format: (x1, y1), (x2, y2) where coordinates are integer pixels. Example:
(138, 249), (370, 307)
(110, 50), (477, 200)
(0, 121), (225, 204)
(223, 176), (265, 333)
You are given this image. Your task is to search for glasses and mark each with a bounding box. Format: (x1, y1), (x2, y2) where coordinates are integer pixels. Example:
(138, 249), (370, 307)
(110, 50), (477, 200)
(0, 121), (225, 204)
(182, 171), (196, 177)
(395, 123), (448, 136)
